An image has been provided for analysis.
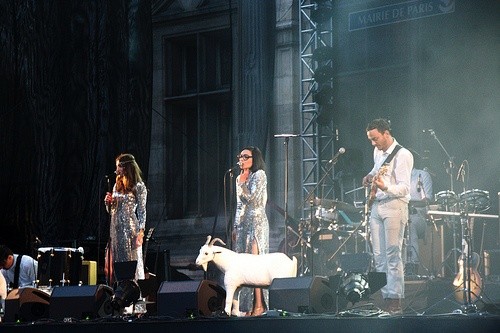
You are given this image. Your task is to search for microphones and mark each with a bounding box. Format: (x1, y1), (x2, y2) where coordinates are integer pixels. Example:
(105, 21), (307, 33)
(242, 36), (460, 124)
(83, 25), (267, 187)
(105, 171), (119, 178)
(228, 162), (243, 173)
(457, 162), (464, 180)
(417, 174), (422, 192)
(329, 148), (345, 163)
(336, 128), (339, 141)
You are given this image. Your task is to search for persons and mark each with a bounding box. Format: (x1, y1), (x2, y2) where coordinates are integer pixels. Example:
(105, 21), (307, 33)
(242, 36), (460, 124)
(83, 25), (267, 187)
(104, 153), (145, 319)
(0, 247), (38, 293)
(231, 147), (270, 317)
(403, 147), (433, 283)
(362, 118), (415, 304)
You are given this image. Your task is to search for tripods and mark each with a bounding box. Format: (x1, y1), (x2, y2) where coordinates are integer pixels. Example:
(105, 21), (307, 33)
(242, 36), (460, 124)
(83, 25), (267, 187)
(420, 168), (500, 315)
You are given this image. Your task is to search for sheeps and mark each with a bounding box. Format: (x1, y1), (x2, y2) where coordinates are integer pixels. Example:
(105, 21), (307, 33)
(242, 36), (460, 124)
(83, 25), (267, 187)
(196, 235), (298, 316)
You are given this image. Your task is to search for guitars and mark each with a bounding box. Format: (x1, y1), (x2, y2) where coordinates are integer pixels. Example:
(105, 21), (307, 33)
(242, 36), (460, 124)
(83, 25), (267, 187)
(366, 165), (389, 207)
(453, 215), (485, 303)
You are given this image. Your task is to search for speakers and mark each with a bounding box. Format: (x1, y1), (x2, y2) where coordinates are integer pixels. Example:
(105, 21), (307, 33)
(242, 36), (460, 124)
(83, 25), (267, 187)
(157, 280), (226, 316)
(50, 283), (114, 317)
(268, 277), (337, 313)
(5, 287), (51, 322)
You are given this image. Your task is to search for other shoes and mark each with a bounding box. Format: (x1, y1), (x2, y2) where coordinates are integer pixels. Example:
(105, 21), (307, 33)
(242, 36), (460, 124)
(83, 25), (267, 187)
(388, 298), (402, 316)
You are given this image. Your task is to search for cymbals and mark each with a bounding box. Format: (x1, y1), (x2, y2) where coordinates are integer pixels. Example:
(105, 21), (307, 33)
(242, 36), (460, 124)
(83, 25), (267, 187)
(312, 198), (356, 224)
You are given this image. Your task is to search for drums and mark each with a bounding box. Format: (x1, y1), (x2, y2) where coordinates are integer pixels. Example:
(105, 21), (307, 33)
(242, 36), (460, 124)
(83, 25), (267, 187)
(35, 247), (85, 287)
(456, 189), (492, 213)
(280, 228), (371, 278)
(434, 190), (458, 209)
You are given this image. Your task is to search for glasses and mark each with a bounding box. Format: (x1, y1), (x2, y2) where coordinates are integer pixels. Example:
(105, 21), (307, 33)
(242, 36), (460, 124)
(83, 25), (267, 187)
(236, 154), (253, 160)
